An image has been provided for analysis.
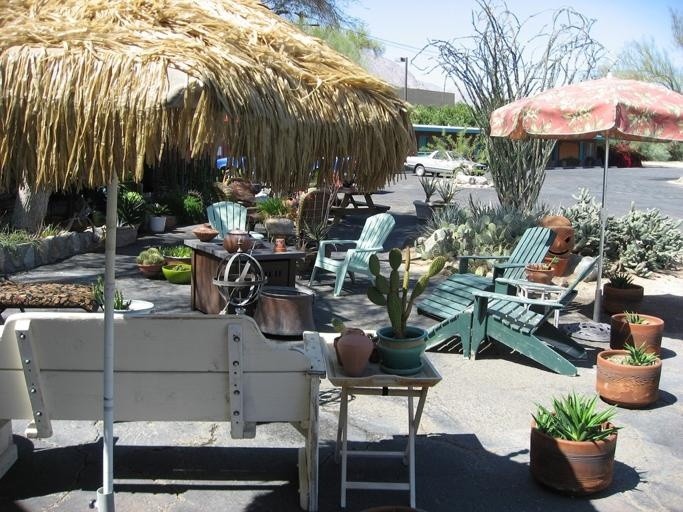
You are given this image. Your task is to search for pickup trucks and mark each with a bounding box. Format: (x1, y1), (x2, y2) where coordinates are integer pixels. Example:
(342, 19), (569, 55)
(405, 149), (487, 180)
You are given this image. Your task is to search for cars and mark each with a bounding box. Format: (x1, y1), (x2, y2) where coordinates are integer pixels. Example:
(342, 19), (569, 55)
(216, 154), (255, 172)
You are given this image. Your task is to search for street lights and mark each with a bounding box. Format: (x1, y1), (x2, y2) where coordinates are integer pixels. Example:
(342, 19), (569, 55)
(398, 54), (409, 101)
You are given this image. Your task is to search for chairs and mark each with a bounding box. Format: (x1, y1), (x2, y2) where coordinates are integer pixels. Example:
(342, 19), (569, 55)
(214, 182), (233, 201)
(206, 201), (247, 239)
(310, 215), (396, 296)
(417, 227), (556, 322)
(294, 190), (330, 247)
(226, 178), (263, 221)
(423, 254), (601, 377)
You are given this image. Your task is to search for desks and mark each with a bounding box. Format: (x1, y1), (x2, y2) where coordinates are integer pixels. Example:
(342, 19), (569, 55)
(516, 283), (560, 328)
(185, 239), (305, 314)
(254, 222), (296, 233)
(319, 331), (439, 508)
(333, 188), (393, 225)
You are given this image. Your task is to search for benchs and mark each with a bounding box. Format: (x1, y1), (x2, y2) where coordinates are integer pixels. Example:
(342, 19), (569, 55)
(348, 199), (391, 212)
(0, 283), (98, 323)
(333, 206), (386, 225)
(329, 204), (340, 213)
(0, 312), (327, 512)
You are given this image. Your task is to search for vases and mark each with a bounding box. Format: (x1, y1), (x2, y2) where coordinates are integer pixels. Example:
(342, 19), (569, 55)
(338, 328), (373, 377)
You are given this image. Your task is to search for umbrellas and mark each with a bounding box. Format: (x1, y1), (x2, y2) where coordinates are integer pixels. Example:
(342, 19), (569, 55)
(490, 73), (683, 344)
(0, 0), (418, 512)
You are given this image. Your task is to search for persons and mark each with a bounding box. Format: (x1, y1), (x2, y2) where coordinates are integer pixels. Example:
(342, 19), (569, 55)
(614, 141), (634, 166)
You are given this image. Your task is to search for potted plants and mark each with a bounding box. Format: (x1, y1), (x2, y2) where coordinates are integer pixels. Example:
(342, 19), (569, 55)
(603, 273), (643, 311)
(136, 248), (164, 277)
(526, 256), (559, 284)
(257, 195), (288, 218)
(92, 276), (154, 314)
(162, 264), (191, 283)
(366, 248), (446, 374)
(116, 192), (145, 248)
(414, 176), (440, 219)
(428, 176), (461, 211)
(530, 389), (625, 496)
(333, 319), (346, 366)
(150, 202), (169, 232)
(165, 211), (176, 231)
(597, 342), (662, 408)
(164, 245), (192, 264)
(609, 311), (664, 355)
